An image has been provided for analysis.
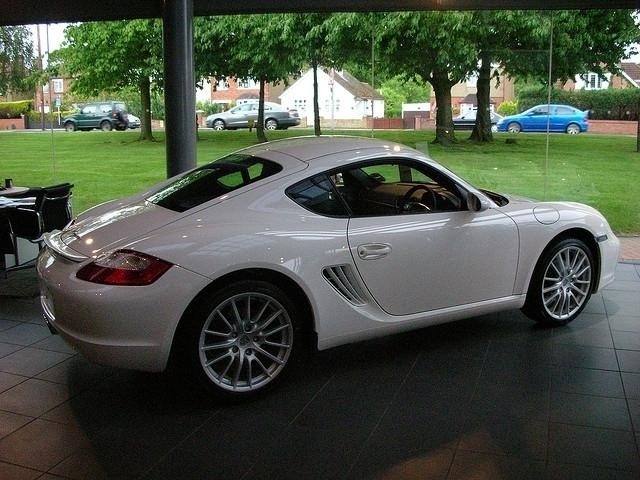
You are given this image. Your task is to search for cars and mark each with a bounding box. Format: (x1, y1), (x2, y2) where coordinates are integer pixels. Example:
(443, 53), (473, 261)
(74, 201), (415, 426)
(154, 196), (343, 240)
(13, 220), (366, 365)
(204, 102), (301, 130)
(496, 104), (590, 134)
(453, 110), (502, 131)
(36, 134), (621, 402)
(127, 113), (142, 129)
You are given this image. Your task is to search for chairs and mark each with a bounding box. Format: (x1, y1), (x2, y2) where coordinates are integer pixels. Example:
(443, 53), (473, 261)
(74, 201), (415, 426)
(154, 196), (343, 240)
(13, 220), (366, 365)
(0, 182), (74, 278)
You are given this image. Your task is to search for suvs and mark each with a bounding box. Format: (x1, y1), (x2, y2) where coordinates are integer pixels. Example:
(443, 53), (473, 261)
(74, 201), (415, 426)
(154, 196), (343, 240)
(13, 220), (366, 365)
(61, 101), (129, 132)
